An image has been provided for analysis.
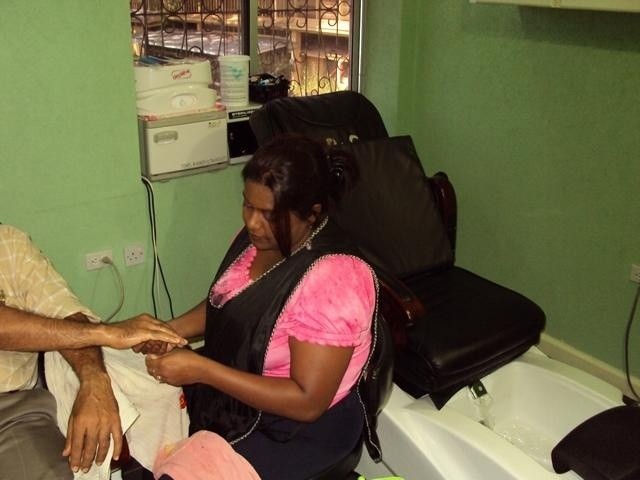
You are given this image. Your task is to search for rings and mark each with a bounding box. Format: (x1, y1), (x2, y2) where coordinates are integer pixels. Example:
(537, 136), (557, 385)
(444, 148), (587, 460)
(156, 374), (164, 381)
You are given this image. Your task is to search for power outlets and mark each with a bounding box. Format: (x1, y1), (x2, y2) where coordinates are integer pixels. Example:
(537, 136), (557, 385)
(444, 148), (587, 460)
(86, 250), (113, 271)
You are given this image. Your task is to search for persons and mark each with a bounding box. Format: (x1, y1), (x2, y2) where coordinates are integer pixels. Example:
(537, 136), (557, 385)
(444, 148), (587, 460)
(0, 222), (190, 480)
(130, 138), (385, 480)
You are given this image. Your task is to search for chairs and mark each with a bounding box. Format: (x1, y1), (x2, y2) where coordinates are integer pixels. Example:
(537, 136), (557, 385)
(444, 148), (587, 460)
(247, 88), (546, 394)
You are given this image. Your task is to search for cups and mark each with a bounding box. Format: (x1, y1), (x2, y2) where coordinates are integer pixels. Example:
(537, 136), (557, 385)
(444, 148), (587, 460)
(216, 53), (251, 108)
